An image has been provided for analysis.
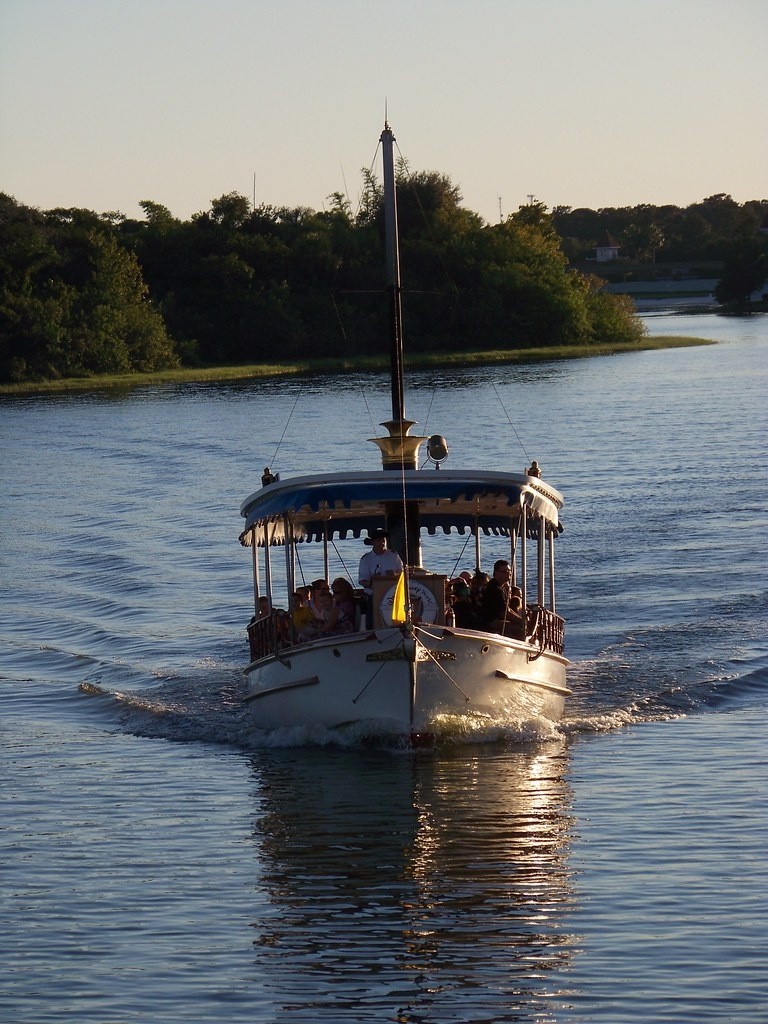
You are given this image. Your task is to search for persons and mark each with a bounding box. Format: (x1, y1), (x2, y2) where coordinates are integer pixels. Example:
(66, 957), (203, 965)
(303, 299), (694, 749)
(447, 560), (531, 638)
(359, 531), (403, 630)
(251, 578), (355, 659)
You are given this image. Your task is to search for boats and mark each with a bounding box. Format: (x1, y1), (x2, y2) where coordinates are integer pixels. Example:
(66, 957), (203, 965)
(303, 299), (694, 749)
(237, 83), (567, 744)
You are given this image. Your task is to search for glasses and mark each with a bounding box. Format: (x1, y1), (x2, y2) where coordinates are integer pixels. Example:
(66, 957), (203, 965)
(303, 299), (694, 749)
(498, 568), (512, 576)
(333, 590), (347, 596)
(320, 587), (329, 593)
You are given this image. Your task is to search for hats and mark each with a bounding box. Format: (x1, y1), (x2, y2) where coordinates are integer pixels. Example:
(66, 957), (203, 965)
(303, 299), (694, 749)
(364, 530), (391, 545)
